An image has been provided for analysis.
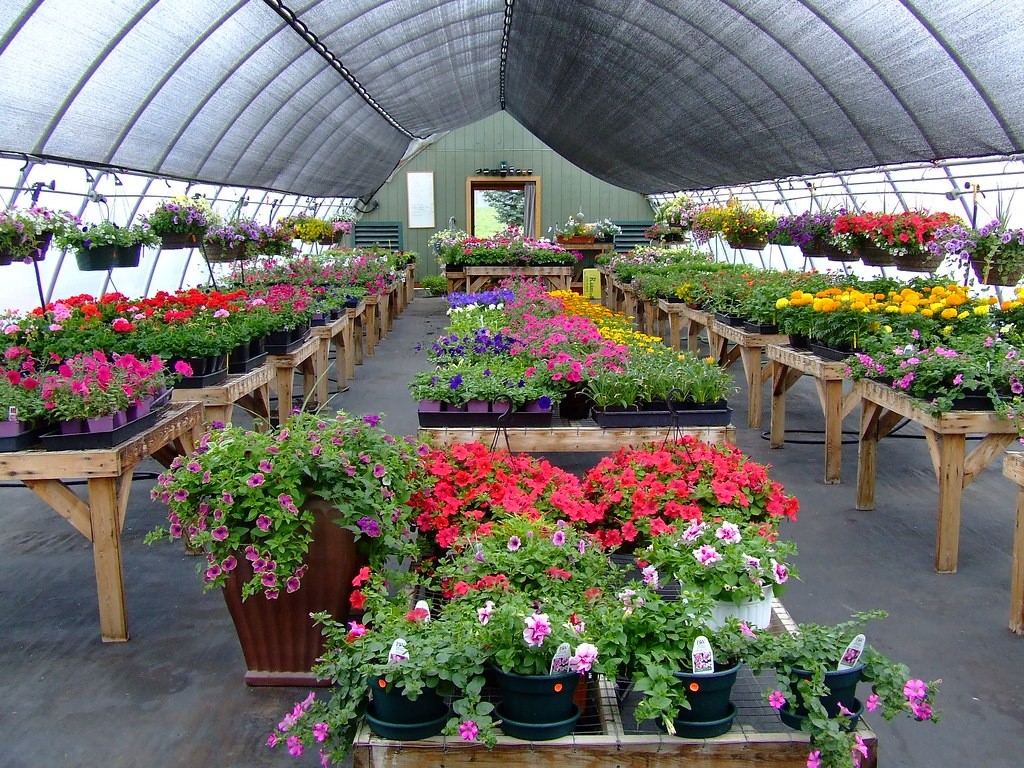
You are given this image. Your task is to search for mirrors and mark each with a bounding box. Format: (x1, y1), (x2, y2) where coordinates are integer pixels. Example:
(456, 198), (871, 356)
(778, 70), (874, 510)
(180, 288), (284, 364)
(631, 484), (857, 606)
(406, 172), (435, 228)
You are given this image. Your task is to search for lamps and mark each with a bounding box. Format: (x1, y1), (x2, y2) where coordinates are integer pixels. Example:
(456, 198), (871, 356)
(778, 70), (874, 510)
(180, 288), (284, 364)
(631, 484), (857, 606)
(475, 161), (533, 176)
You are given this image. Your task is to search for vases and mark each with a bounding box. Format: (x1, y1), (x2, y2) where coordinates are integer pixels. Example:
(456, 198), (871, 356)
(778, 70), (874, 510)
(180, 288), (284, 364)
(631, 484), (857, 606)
(419, 378), (591, 420)
(2, 197), (354, 271)
(779, 684), (866, 731)
(486, 661), (582, 741)
(612, 196), (1022, 407)
(554, 233), (594, 245)
(363, 669), (454, 741)
(654, 659), (744, 739)
(0, 246), (416, 438)
(683, 582), (774, 634)
(224, 500), (362, 689)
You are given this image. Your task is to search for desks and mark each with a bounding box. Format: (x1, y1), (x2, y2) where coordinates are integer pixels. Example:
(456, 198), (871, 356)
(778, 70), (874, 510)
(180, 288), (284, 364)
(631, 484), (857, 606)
(366, 291), (382, 355)
(463, 266), (575, 293)
(267, 331), (321, 429)
(0, 400), (207, 640)
(764, 342), (863, 487)
(712, 320), (790, 429)
(852, 376), (1023, 575)
(446, 272), (466, 295)
(352, 589), (877, 768)
(310, 316), (352, 407)
(1001, 451), (1024, 636)
(168, 359), (277, 428)
